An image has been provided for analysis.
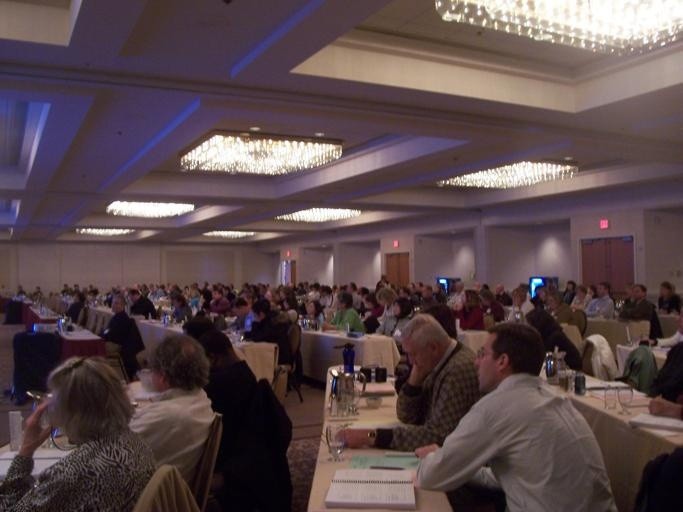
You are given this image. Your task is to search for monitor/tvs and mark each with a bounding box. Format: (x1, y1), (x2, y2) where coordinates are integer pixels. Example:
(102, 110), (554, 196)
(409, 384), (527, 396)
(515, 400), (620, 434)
(529, 276), (558, 299)
(437, 278), (461, 295)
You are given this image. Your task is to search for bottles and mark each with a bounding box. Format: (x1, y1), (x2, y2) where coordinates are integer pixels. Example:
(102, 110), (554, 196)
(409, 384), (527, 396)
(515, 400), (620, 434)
(332, 342), (354, 380)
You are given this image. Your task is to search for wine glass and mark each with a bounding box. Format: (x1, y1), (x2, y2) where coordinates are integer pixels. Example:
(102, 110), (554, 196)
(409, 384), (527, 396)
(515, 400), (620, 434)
(323, 423), (347, 463)
(616, 380), (633, 415)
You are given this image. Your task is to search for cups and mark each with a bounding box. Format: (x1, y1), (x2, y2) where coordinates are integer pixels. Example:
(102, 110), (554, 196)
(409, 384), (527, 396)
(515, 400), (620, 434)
(374, 366), (386, 382)
(545, 350), (568, 385)
(604, 386), (617, 408)
(6, 409), (22, 451)
(359, 367), (372, 383)
(556, 367), (586, 394)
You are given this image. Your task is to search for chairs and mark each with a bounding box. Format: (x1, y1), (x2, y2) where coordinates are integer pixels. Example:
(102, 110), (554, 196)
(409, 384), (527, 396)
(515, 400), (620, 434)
(190, 412), (223, 510)
(132, 465), (188, 511)
(106, 354), (128, 383)
(288, 323), (304, 403)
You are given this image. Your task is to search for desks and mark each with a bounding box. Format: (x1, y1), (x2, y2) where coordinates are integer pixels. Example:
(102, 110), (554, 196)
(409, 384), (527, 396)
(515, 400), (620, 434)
(537, 312), (682, 448)
(1, 380), (162, 481)
(504, 305), (518, 314)
(307, 365), (452, 510)
(137, 316), (278, 349)
(155, 305), (171, 313)
(457, 330), (490, 352)
(22, 299), (115, 359)
(301, 329), (393, 343)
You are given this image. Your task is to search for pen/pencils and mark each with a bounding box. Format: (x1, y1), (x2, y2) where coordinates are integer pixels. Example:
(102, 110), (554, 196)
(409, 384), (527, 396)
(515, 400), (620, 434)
(370, 466), (406, 471)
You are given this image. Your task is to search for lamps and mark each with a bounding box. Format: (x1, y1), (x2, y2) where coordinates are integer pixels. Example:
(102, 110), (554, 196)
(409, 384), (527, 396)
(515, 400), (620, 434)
(436, 161), (580, 190)
(434, 1), (683, 57)
(76, 227), (135, 237)
(273, 208), (361, 223)
(106, 200), (195, 219)
(177, 129), (345, 176)
(202, 231), (256, 240)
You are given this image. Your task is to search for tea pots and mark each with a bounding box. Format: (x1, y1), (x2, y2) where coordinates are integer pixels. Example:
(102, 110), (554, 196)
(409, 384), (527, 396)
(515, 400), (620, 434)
(328, 367), (366, 416)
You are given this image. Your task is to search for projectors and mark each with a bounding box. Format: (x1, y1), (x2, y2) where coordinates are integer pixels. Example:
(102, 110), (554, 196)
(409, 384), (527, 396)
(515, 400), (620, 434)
(32, 322), (56, 334)
